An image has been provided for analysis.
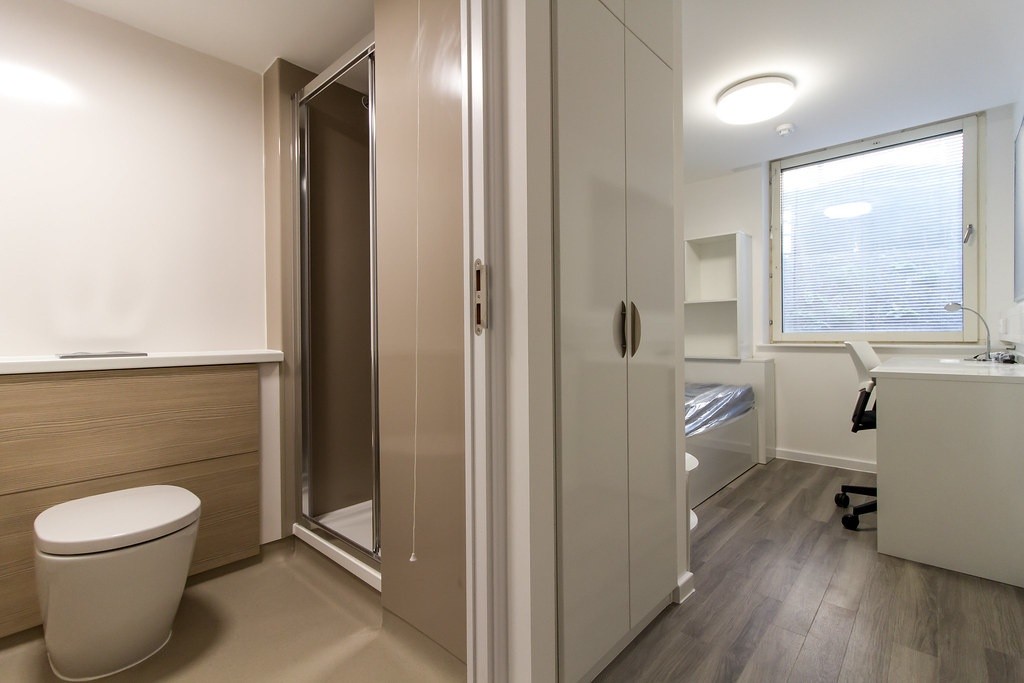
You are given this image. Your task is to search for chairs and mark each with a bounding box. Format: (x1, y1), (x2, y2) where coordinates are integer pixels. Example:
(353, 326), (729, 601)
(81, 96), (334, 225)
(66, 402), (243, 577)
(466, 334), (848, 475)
(834, 340), (881, 530)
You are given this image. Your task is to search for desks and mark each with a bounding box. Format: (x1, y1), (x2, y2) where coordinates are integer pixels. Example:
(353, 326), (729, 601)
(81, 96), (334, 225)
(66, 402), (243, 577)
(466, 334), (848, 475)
(871, 353), (1024, 587)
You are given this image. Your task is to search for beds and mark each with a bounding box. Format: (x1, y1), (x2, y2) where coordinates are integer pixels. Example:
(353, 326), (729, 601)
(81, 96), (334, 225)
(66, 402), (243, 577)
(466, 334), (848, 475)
(686, 356), (775, 511)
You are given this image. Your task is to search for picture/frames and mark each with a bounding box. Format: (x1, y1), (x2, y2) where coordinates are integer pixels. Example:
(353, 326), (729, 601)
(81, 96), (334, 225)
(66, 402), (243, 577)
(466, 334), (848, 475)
(1014, 115), (1024, 303)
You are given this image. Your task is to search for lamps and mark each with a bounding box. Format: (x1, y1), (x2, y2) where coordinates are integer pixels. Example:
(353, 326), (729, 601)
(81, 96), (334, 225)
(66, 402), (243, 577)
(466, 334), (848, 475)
(943, 302), (991, 362)
(716, 72), (800, 128)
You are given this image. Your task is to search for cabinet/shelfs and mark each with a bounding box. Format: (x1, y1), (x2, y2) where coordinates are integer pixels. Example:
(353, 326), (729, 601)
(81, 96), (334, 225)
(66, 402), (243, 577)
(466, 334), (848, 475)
(551, 0), (697, 683)
(682, 230), (753, 360)
